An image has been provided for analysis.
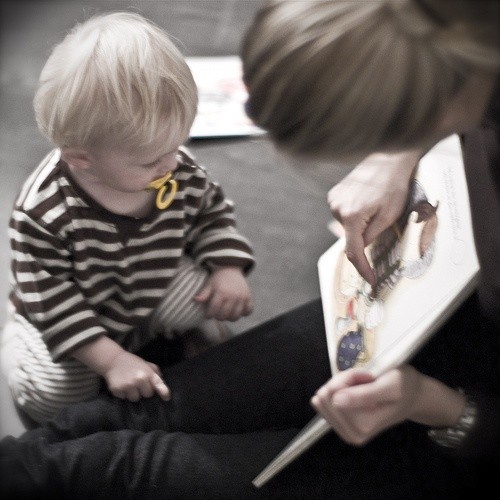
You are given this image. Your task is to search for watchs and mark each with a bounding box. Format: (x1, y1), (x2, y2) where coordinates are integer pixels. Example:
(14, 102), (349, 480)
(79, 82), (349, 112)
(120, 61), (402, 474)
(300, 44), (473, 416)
(428, 401), (479, 452)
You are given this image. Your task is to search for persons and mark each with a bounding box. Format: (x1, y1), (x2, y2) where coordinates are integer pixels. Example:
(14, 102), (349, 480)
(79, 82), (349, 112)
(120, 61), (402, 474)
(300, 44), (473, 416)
(2, 3), (499, 500)
(0, 10), (258, 430)
